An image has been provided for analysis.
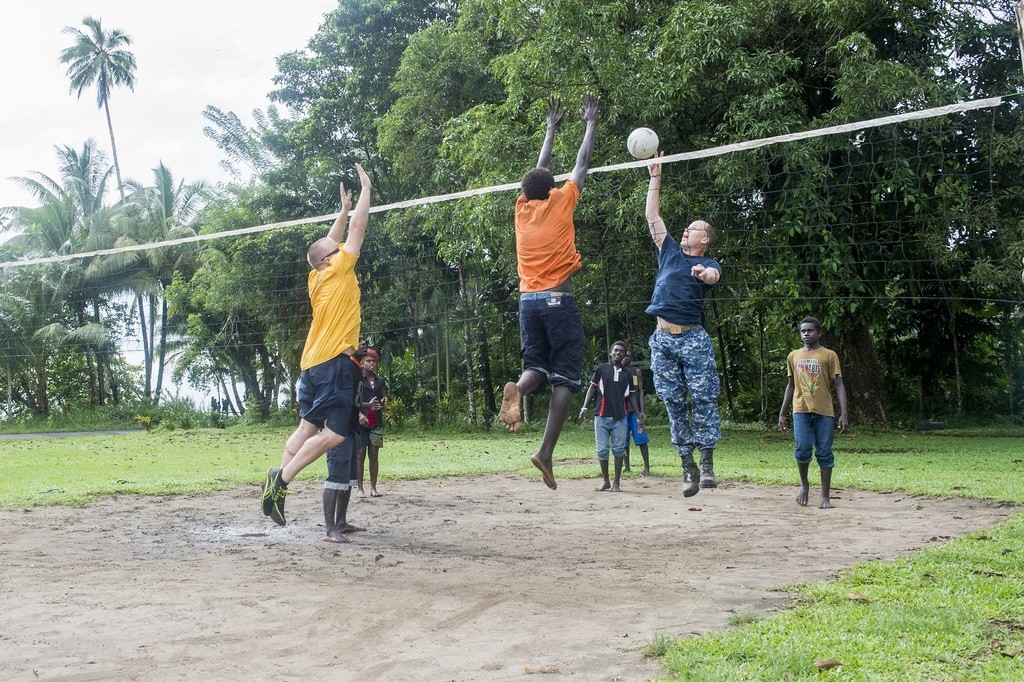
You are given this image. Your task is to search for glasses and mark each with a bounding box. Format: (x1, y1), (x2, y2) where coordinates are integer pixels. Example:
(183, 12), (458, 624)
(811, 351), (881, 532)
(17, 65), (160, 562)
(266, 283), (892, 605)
(320, 240), (343, 262)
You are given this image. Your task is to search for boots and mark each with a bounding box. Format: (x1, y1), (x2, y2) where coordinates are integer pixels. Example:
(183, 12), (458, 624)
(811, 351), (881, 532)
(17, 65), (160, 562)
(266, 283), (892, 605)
(700, 449), (717, 487)
(681, 454), (700, 497)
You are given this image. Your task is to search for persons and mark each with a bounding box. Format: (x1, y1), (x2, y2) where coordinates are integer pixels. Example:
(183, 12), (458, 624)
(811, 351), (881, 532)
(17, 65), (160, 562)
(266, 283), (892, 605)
(211, 396), (230, 417)
(499, 91), (603, 490)
(258, 162), (371, 528)
(320, 333), (392, 543)
(578, 339), (651, 493)
(780, 317), (848, 509)
(647, 150), (723, 497)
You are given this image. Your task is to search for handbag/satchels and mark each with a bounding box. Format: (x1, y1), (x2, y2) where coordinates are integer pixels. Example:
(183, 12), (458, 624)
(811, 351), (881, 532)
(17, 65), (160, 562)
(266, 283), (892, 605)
(370, 429), (383, 447)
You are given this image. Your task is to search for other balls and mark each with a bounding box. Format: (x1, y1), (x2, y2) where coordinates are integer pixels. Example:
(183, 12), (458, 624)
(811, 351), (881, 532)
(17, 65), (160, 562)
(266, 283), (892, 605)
(626, 127), (659, 159)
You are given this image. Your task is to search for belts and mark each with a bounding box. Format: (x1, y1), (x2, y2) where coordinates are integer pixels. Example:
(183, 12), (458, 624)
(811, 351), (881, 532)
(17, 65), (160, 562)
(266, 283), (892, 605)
(660, 325), (698, 334)
(520, 291), (574, 301)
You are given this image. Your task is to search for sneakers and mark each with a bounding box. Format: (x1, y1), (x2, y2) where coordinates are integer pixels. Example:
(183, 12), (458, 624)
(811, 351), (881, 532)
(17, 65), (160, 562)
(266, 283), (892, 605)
(262, 483), (286, 526)
(261, 464), (288, 516)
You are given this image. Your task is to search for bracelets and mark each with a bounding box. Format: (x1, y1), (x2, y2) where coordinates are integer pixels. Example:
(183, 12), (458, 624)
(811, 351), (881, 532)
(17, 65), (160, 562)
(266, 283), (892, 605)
(650, 173), (662, 179)
